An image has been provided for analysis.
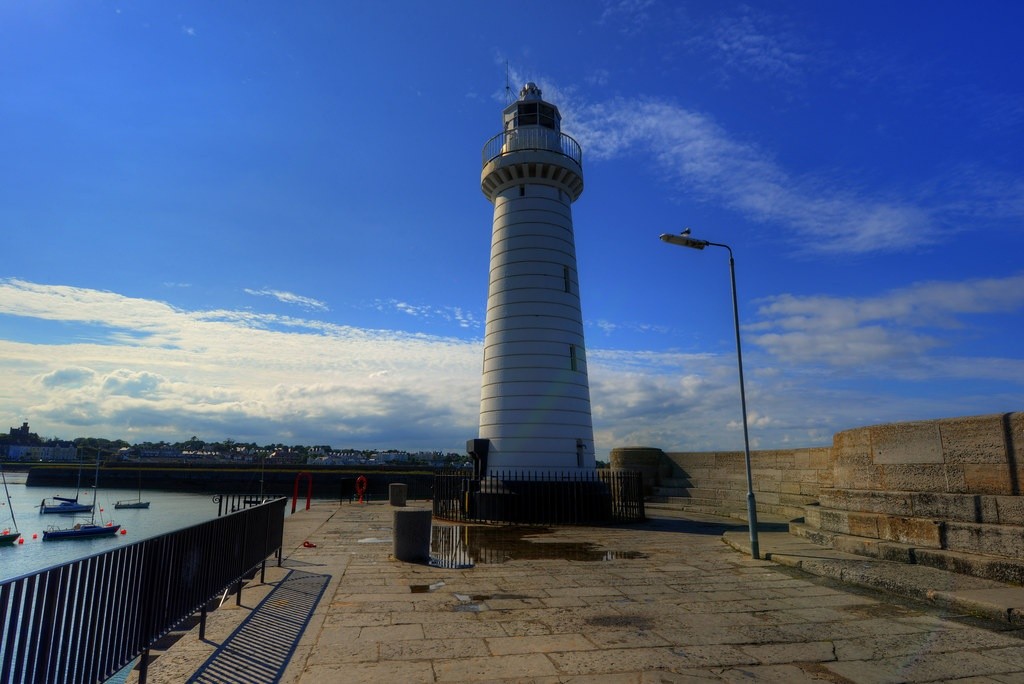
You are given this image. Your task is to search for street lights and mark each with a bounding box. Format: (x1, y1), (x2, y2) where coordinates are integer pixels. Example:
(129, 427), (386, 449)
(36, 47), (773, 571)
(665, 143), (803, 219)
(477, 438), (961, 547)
(660, 228), (761, 558)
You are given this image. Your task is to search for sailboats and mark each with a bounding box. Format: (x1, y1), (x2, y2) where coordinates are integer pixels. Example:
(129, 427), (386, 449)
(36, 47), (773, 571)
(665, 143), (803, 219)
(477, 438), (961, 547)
(1, 472), (22, 545)
(38, 449), (94, 515)
(41, 448), (123, 542)
(115, 466), (151, 510)
